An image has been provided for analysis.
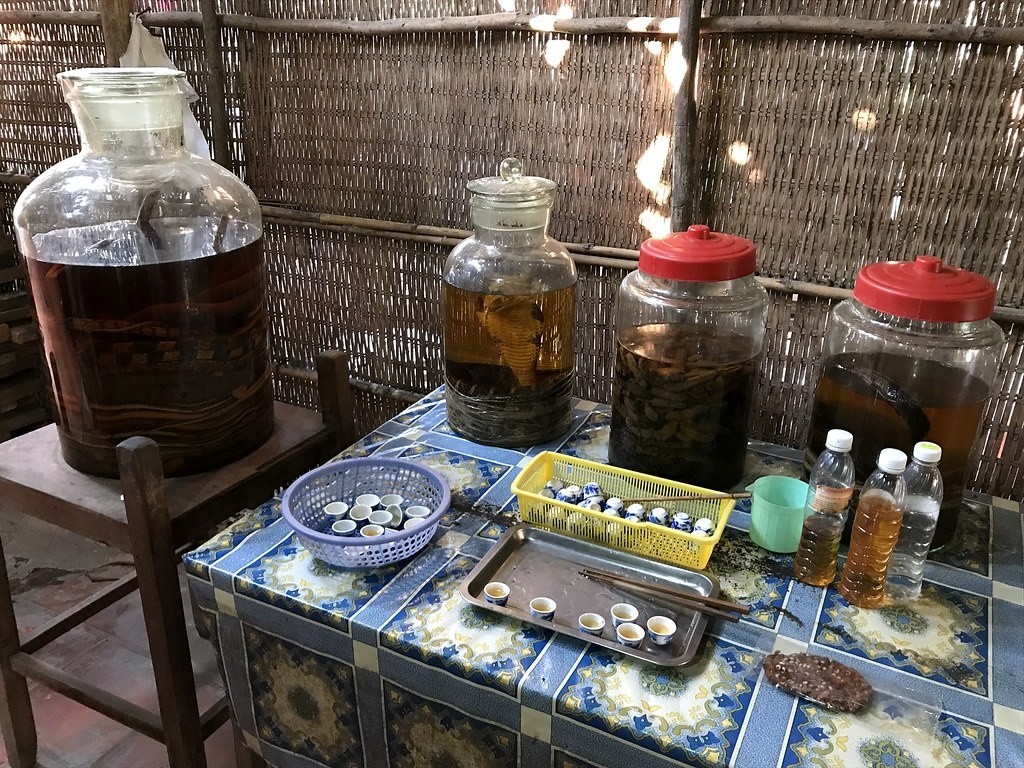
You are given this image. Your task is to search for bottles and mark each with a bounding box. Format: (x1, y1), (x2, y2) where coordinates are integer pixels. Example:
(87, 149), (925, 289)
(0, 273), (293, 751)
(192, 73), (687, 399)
(839, 447), (908, 609)
(795, 429), (855, 586)
(884, 441), (943, 599)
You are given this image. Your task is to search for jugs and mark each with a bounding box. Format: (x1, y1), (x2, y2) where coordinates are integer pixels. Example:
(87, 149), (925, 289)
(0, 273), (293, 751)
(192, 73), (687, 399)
(745, 475), (812, 552)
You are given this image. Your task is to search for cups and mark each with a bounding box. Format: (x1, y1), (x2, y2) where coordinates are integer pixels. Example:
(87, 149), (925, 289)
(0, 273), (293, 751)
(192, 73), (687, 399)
(647, 616), (677, 646)
(611, 603), (639, 627)
(484, 582), (510, 606)
(325, 495), (430, 556)
(616, 623), (645, 650)
(526, 480), (716, 552)
(578, 613), (606, 638)
(529, 597), (556, 622)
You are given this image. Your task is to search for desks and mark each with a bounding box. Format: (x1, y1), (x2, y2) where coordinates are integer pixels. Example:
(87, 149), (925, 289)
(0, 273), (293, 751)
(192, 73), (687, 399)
(0, 349), (350, 768)
(182, 383), (1024, 768)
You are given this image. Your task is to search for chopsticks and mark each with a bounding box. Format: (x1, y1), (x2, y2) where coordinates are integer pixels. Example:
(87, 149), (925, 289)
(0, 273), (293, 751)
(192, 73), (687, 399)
(578, 569), (751, 621)
